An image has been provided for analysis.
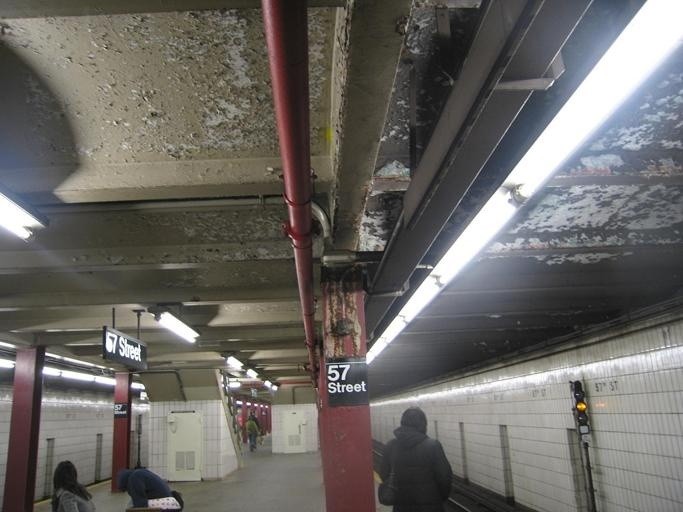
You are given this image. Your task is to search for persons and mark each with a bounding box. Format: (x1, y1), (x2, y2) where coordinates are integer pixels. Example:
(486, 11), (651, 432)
(247, 411), (260, 432)
(376, 404), (454, 510)
(117, 467), (182, 512)
(51, 459), (95, 511)
(245, 415), (260, 451)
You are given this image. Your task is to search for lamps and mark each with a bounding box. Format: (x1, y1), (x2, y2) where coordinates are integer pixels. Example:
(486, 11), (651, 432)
(0, 183), (49, 246)
(147, 305), (201, 345)
(222, 350), (280, 392)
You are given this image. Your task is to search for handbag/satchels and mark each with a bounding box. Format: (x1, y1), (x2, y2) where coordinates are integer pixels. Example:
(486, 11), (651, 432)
(171, 491), (184, 507)
(378, 473), (399, 506)
(147, 496), (182, 511)
